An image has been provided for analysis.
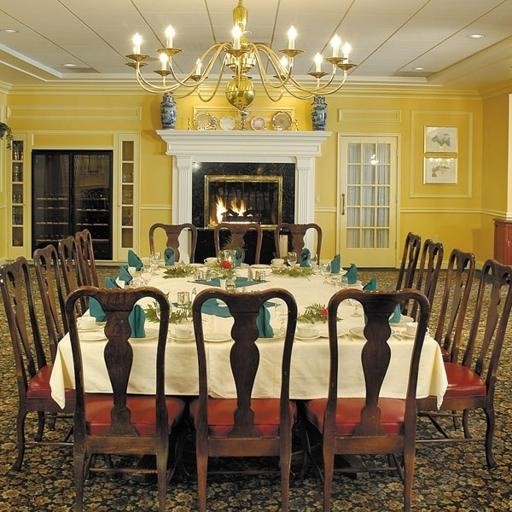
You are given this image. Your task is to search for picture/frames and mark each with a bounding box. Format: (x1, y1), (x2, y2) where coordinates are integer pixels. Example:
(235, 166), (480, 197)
(422, 125), (460, 155)
(423, 157), (459, 185)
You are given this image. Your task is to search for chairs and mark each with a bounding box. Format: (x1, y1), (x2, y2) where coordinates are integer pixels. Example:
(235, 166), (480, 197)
(392, 232), (422, 316)
(32, 244), (81, 430)
(386, 258), (511, 476)
(434, 248), (477, 430)
(75, 229), (98, 313)
(306, 288), (430, 512)
(411, 239), (444, 323)
(274, 222), (322, 265)
(1, 256), (119, 482)
(214, 222), (263, 265)
(149, 223), (198, 265)
(189, 287), (297, 510)
(64, 287), (185, 511)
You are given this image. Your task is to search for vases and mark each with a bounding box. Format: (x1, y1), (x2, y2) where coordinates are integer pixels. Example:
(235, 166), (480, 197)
(218, 278), (236, 288)
(311, 95), (328, 130)
(159, 93), (178, 129)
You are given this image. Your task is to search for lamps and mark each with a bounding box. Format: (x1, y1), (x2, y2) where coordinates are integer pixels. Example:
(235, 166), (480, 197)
(126, 0), (356, 115)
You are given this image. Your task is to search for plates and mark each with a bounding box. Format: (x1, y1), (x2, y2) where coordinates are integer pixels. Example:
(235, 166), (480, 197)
(251, 264), (273, 275)
(76, 315), (430, 343)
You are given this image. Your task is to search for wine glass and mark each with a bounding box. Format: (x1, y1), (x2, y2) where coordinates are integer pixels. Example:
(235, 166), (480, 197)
(287, 248), (348, 290)
(131, 248), (161, 287)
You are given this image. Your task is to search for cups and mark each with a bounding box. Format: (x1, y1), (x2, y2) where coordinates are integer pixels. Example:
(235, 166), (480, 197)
(204, 257), (217, 264)
(296, 324), (319, 337)
(76, 317), (96, 330)
(406, 323), (419, 335)
(271, 259), (285, 266)
(173, 325), (193, 339)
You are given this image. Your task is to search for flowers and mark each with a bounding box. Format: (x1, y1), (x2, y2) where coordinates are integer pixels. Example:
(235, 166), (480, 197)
(297, 303), (337, 324)
(206, 254), (234, 279)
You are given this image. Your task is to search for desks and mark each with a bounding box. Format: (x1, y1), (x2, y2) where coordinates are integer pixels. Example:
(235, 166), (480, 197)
(57, 263), (439, 489)
